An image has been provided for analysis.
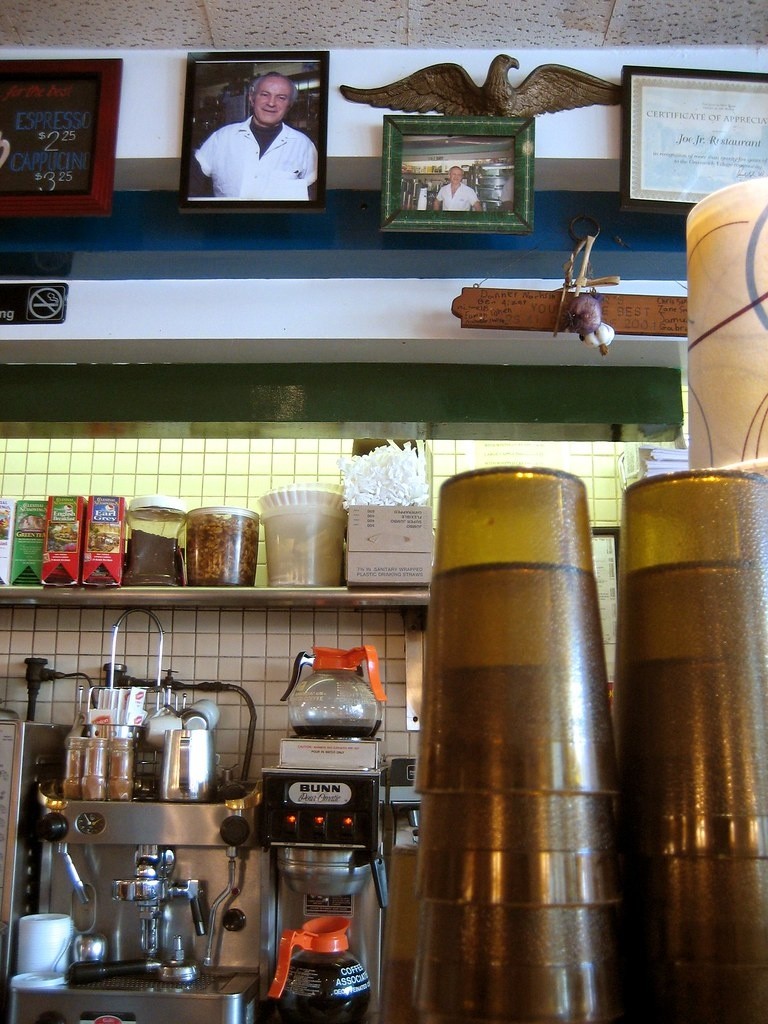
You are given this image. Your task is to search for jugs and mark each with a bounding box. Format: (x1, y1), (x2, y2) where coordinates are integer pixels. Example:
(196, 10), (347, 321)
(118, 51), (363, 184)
(280, 643), (383, 738)
(258, 914), (372, 1024)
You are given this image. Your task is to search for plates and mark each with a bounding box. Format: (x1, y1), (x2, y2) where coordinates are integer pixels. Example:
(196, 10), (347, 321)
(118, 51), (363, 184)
(12, 913), (74, 989)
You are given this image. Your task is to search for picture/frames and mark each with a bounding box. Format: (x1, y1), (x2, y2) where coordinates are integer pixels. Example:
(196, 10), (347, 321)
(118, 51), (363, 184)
(176, 51), (330, 212)
(378, 114), (536, 236)
(0, 58), (124, 219)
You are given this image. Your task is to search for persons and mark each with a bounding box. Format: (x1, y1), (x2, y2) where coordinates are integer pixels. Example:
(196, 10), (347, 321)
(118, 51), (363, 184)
(188, 71), (317, 200)
(433, 166), (483, 212)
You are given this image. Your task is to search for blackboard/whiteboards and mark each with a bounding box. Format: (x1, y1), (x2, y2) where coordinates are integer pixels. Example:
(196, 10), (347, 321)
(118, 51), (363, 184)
(0, 57), (124, 219)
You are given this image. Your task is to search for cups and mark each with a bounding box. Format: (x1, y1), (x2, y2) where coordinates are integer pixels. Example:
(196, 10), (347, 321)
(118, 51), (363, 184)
(145, 697), (221, 750)
(160, 728), (214, 802)
(383, 465), (768, 1024)
(681, 177), (768, 466)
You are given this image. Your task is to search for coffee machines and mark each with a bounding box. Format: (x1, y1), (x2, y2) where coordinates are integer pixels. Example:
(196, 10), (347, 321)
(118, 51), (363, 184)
(252, 768), (379, 1024)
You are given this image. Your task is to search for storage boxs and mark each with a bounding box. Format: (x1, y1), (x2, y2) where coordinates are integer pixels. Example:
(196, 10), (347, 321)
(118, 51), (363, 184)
(347, 439), (432, 585)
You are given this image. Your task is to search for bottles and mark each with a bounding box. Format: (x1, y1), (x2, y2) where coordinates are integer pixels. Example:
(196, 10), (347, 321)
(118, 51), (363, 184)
(106, 737), (135, 800)
(63, 735), (87, 800)
(81, 738), (106, 800)
